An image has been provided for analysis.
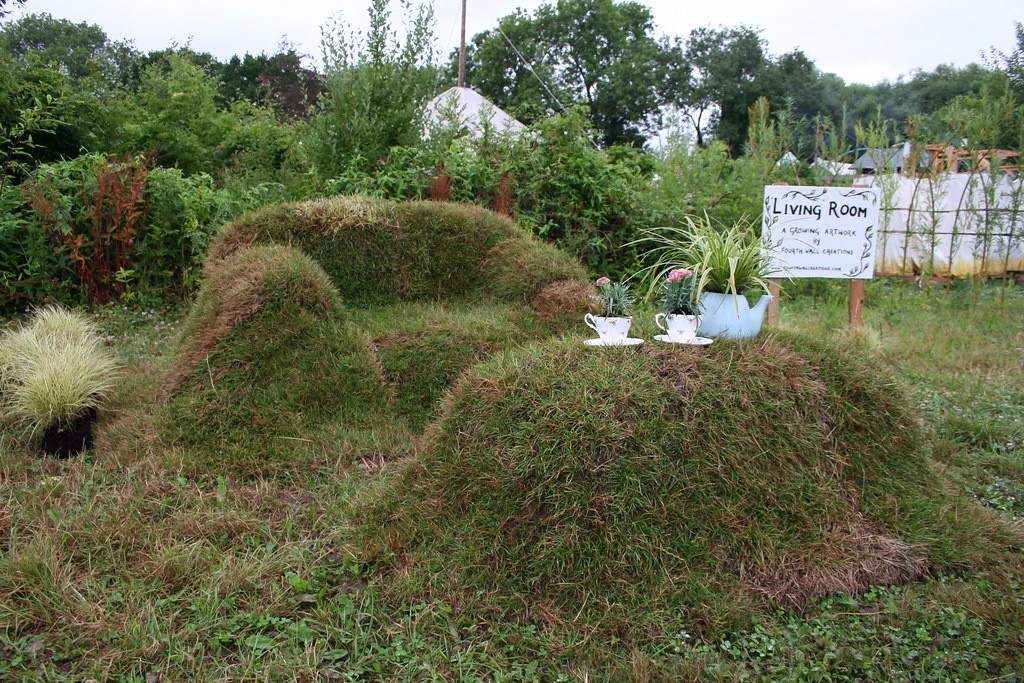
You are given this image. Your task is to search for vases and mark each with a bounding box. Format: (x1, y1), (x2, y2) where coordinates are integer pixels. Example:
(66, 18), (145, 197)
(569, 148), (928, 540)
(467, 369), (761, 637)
(583, 312), (644, 347)
(655, 313), (714, 345)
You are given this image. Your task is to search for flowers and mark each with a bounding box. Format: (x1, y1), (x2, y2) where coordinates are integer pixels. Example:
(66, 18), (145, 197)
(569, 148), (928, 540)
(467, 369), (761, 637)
(656, 269), (706, 331)
(584, 276), (631, 321)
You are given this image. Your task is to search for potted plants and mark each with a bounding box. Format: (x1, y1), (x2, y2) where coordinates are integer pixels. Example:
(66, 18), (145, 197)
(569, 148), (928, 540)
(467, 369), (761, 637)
(618, 207), (800, 339)
(0, 304), (123, 459)
